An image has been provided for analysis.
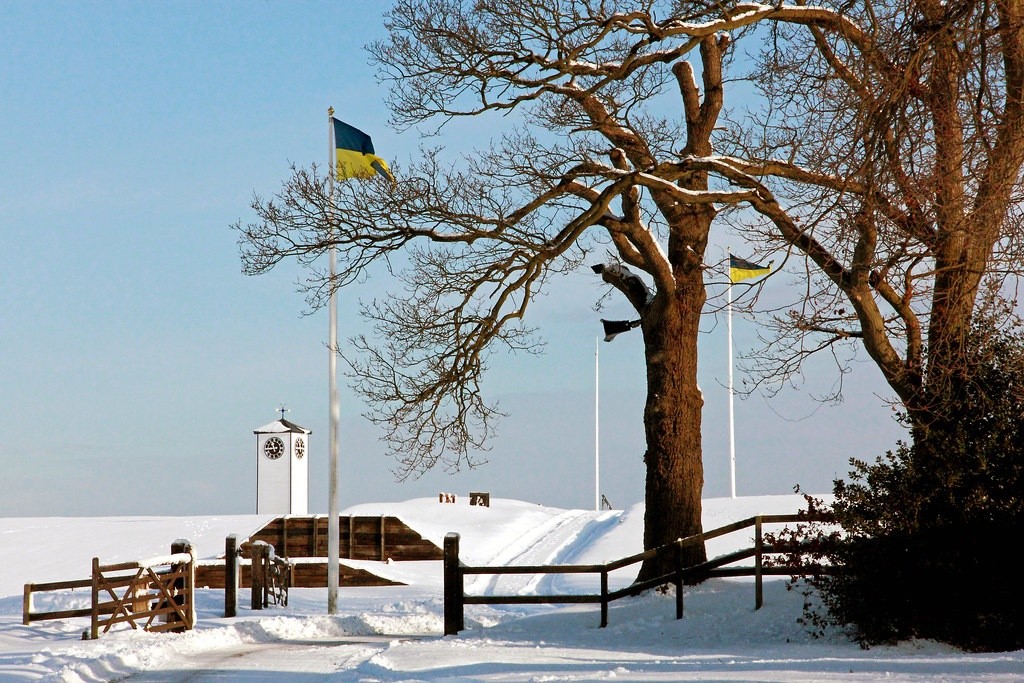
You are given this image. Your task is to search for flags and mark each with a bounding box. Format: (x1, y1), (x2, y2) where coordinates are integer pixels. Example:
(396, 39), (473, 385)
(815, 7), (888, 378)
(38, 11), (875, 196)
(332, 118), (395, 184)
(730, 253), (773, 282)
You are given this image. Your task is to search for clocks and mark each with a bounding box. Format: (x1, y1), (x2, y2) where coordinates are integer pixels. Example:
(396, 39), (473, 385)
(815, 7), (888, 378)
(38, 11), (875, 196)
(293, 437), (305, 459)
(264, 437), (285, 459)
